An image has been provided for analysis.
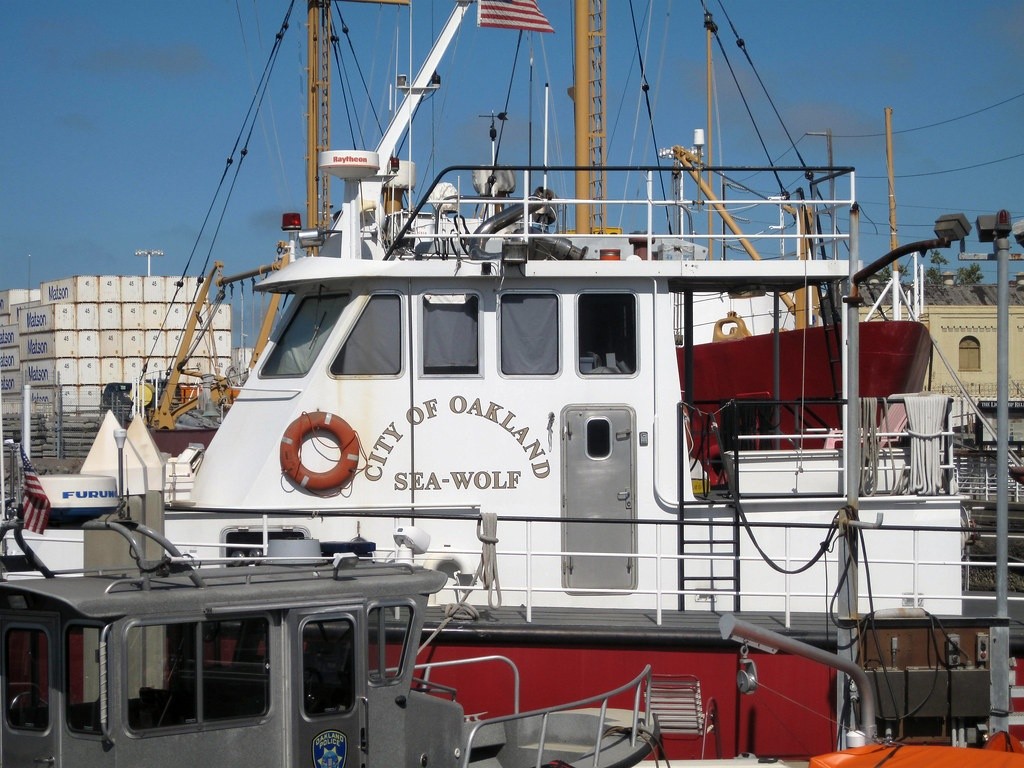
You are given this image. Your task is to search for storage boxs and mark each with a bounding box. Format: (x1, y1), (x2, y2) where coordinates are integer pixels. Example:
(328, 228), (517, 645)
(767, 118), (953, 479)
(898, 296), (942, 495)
(0, 275), (231, 418)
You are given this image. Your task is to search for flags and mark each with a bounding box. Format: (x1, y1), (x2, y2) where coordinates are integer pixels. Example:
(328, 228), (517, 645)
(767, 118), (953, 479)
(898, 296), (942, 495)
(478, 0), (555, 32)
(20, 446), (49, 534)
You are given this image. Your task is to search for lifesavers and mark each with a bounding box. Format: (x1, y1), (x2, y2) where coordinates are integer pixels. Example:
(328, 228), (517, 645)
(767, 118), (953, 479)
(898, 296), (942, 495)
(279, 410), (360, 495)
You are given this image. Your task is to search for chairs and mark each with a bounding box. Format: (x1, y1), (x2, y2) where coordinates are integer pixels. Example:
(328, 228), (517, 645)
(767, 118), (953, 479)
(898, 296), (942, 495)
(823, 402), (908, 450)
(643, 675), (721, 762)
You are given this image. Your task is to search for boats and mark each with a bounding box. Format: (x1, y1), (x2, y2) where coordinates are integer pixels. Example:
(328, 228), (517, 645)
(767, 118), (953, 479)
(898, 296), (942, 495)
(2, 0), (1023, 768)
